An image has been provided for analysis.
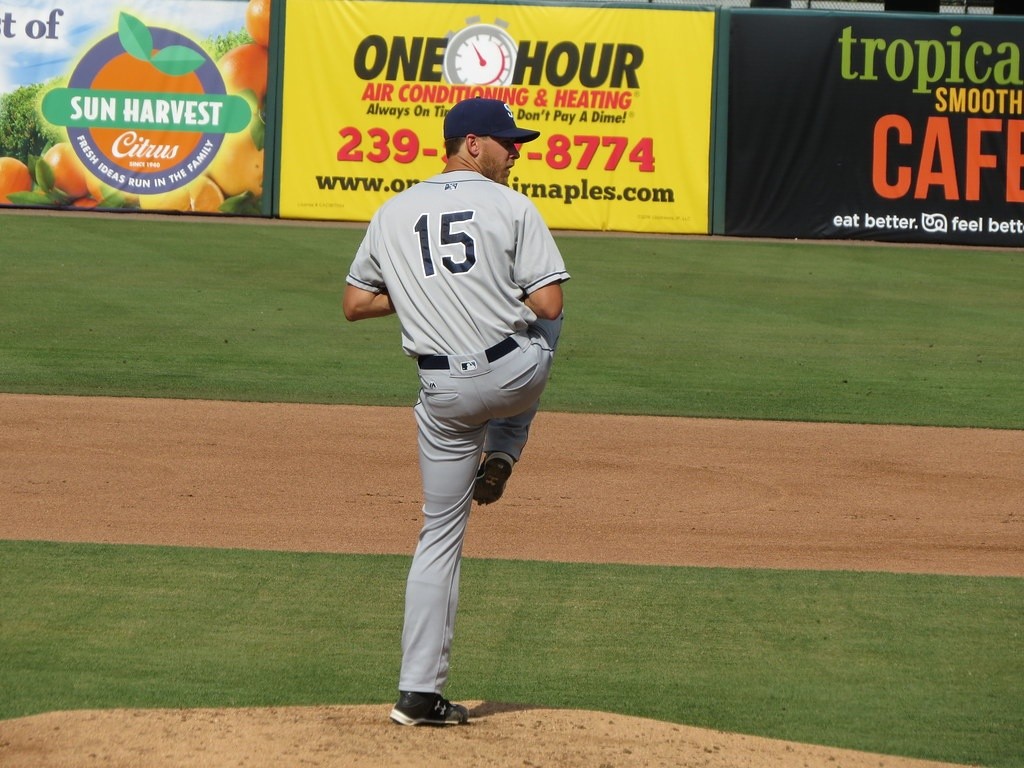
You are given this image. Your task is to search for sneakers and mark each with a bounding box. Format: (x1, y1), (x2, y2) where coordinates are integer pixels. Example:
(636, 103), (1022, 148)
(472, 451), (516, 503)
(390, 692), (468, 727)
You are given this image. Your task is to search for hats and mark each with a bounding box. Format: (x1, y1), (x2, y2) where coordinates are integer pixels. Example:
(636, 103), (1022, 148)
(444, 96), (540, 144)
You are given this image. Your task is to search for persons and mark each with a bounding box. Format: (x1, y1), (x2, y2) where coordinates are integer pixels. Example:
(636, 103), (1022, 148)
(341, 95), (571, 728)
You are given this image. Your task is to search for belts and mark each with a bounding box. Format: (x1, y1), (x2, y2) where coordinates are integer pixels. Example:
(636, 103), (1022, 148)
(417, 337), (518, 370)
